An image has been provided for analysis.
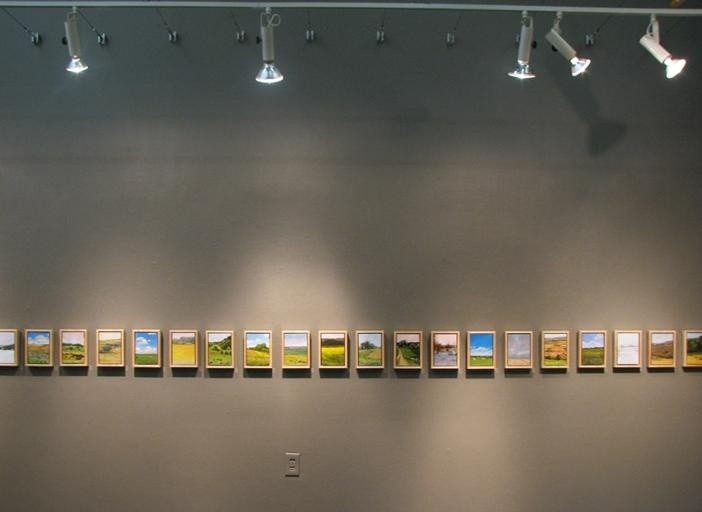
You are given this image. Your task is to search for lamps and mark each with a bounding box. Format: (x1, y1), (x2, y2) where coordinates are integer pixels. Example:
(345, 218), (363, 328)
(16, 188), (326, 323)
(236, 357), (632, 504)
(545, 11), (591, 76)
(508, 10), (536, 80)
(640, 13), (686, 79)
(64, 6), (88, 73)
(255, 7), (284, 84)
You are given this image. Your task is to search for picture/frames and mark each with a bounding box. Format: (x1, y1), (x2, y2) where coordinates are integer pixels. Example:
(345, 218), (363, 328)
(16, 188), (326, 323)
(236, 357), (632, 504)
(541, 331), (569, 368)
(647, 330), (677, 368)
(318, 330), (349, 368)
(613, 330), (643, 368)
(681, 329), (702, 367)
(132, 330), (162, 368)
(505, 330), (533, 369)
(169, 330), (199, 368)
(281, 331), (311, 369)
(467, 331), (496, 369)
(1, 329), (20, 367)
(578, 330), (608, 368)
(24, 330), (54, 367)
(204, 330), (234, 369)
(355, 330), (384, 369)
(430, 330), (460, 370)
(59, 330), (89, 367)
(393, 330), (423, 369)
(96, 329), (125, 367)
(243, 331), (272, 368)
